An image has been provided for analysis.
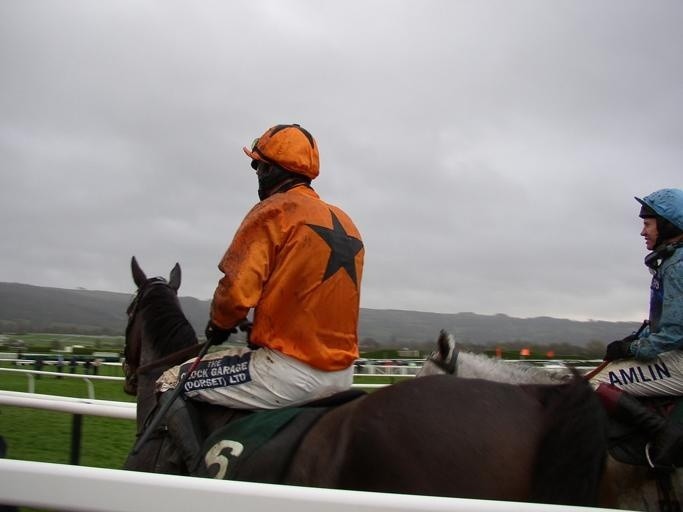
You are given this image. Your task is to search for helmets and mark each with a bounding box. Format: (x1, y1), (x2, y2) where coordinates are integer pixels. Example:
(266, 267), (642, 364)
(633, 187), (683, 232)
(243, 122), (321, 181)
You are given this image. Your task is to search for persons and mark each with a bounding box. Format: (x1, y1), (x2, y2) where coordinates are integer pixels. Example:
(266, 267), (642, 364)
(33, 358), (101, 380)
(581, 188), (681, 467)
(152, 124), (365, 478)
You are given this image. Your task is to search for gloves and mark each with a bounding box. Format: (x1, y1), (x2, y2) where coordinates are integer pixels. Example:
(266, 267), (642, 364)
(204, 320), (238, 345)
(625, 334), (638, 343)
(607, 339), (631, 358)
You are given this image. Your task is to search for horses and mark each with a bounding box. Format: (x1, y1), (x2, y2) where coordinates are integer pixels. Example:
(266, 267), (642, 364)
(413, 329), (682, 512)
(120, 255), (609, 509)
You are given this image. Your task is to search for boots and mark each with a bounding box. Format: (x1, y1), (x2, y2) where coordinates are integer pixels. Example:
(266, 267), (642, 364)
(593, 382), (683, 480)
(164, 403), (207, 477)
